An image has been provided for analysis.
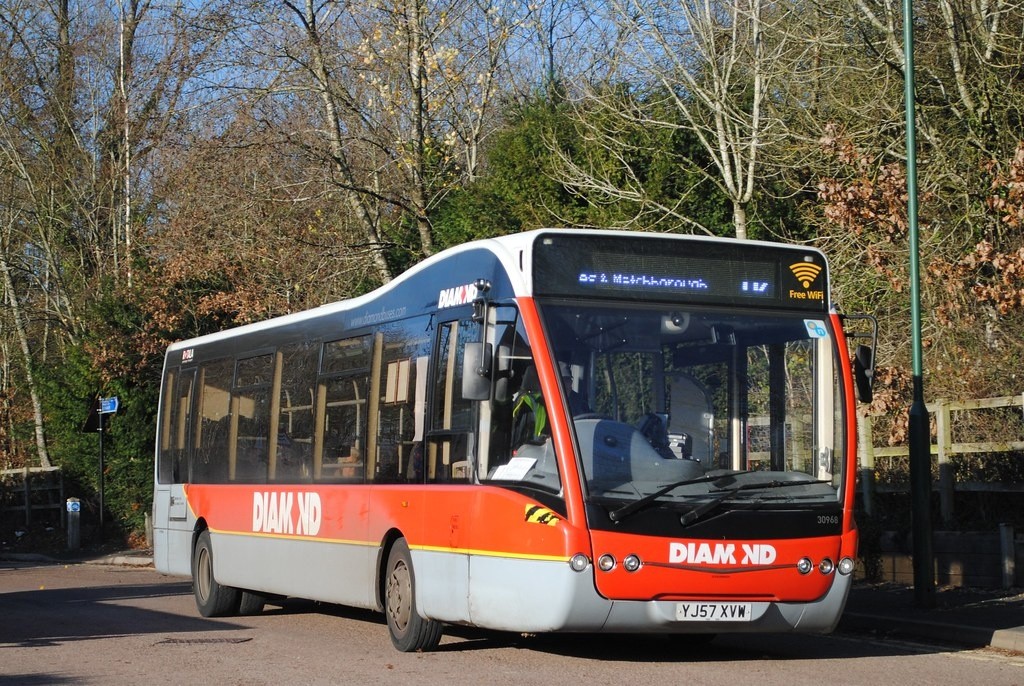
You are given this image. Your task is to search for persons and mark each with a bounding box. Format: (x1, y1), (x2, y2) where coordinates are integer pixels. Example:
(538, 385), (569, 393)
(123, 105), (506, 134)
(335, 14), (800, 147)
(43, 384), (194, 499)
(507, 359), (595, 465)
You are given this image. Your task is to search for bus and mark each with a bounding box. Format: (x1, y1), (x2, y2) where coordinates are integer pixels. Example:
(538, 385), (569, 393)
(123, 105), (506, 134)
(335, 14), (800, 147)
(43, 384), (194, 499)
(146, 226), (879, 661)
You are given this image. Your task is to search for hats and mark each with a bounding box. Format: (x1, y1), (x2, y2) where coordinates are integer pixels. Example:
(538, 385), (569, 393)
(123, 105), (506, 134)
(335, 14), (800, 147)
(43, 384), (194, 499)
(558, 361), (575, 380)
